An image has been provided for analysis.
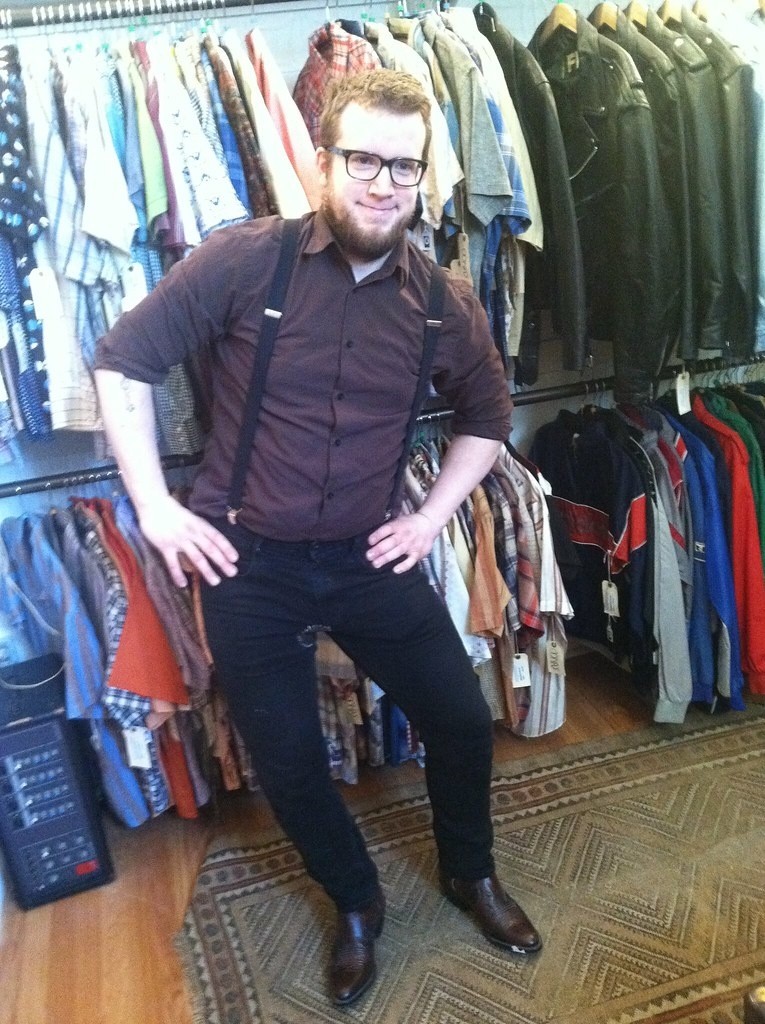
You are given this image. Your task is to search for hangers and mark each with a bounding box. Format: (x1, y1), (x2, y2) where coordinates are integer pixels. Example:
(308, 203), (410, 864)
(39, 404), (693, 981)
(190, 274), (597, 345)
(0, 0), (765, 529)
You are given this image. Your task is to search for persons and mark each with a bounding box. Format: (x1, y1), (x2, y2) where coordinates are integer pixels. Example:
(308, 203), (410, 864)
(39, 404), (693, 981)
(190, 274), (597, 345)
(95, 70), (541, 1007)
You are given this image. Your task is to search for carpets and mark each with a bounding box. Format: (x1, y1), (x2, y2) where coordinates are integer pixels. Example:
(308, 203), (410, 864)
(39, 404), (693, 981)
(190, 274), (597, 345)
(171, 699), (765, 1024)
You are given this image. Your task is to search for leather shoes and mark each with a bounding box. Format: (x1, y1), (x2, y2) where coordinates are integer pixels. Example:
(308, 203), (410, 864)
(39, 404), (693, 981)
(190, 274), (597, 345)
(440, 865), (541, 954)
(330, 883), (385, 1003)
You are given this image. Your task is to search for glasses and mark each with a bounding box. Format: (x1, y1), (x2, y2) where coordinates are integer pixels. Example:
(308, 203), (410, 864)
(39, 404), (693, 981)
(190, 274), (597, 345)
(325, 145), (429, 187)
